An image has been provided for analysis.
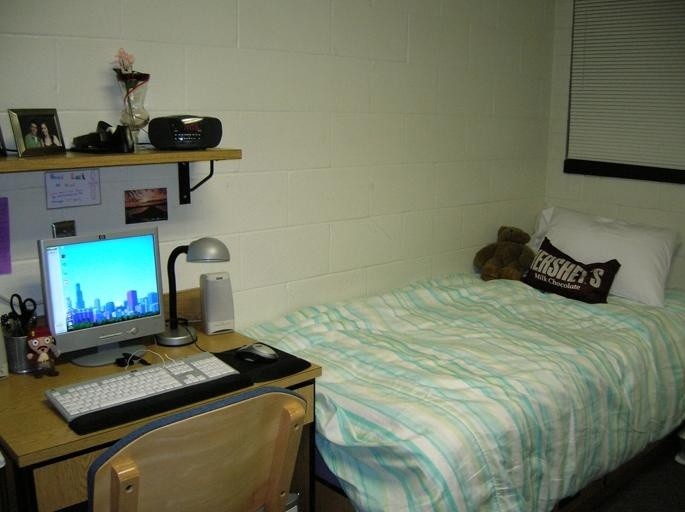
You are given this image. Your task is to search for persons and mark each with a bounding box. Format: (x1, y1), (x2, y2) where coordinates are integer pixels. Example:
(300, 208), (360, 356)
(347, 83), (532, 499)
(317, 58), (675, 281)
(24, 119), (41, 150)
(27, 325), (60, 379)
(40, 120), (59, 147)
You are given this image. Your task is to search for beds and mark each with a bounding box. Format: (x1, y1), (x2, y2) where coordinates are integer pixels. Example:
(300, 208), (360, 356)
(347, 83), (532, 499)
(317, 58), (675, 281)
(239, 274), (685, 512)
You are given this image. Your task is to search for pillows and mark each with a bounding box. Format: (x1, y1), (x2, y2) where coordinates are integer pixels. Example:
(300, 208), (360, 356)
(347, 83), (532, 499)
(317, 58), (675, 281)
(527, 206), (680, 308)
(521, 237), (621, 303)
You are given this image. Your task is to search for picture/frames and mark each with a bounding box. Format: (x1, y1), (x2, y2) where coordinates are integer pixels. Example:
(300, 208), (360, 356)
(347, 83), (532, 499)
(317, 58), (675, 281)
(8, 109), (66, 158)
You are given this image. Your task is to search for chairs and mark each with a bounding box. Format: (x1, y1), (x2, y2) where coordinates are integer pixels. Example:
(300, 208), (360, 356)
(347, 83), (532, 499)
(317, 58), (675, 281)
(87, 386), (308, 510)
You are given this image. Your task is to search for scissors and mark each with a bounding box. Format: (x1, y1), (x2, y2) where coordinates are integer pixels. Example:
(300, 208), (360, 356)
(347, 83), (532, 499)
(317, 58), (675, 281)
(10, 294), (36, 336)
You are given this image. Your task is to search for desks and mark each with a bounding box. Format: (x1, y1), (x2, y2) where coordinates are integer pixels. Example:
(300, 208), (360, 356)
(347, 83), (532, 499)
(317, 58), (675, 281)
(0, 288), (321, 512)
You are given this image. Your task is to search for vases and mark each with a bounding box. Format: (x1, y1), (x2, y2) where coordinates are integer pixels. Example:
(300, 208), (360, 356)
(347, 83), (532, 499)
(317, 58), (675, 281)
(116, 74), (149, 143)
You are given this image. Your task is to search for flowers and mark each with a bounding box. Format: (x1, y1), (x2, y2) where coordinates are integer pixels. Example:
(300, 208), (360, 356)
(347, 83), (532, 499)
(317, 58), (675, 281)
(112, 48), (135, 120)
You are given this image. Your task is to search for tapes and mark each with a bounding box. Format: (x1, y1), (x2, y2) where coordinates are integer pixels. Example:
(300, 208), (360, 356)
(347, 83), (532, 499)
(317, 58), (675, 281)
(105, 125), (116, 134)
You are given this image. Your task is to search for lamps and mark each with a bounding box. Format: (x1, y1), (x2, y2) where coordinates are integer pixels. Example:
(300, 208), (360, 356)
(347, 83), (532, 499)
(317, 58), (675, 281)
(157, 238), (230, 347)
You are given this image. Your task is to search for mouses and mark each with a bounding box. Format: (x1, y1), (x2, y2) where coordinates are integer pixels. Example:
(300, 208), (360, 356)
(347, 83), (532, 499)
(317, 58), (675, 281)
(238, 342), (281, 364)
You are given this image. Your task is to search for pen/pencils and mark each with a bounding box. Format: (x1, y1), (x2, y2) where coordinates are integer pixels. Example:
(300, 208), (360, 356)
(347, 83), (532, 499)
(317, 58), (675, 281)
(1, 312), (40, 337)
(122, 352), (150, 364)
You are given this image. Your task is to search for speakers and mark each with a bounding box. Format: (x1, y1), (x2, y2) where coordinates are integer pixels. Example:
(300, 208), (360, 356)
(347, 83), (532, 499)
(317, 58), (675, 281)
(200, 273), (235, 336)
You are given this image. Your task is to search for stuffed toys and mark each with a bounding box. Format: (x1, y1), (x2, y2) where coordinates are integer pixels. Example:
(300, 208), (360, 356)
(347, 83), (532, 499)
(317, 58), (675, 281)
(473, 224), (534, 280)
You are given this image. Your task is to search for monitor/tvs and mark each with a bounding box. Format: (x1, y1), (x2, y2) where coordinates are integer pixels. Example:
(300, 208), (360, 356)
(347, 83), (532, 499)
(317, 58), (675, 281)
(37, 226), (165, 368)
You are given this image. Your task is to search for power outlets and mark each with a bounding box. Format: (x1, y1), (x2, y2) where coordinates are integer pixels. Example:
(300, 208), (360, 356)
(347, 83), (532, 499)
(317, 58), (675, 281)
(52, 221), (75, 238)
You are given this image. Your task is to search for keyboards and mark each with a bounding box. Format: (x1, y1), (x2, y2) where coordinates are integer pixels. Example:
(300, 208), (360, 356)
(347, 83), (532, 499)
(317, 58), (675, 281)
(44, 351), (240, 422)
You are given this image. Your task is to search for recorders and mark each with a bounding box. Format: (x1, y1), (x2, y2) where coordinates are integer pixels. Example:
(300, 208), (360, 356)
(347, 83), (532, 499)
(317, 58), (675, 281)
(149, 117), (222, 150)
